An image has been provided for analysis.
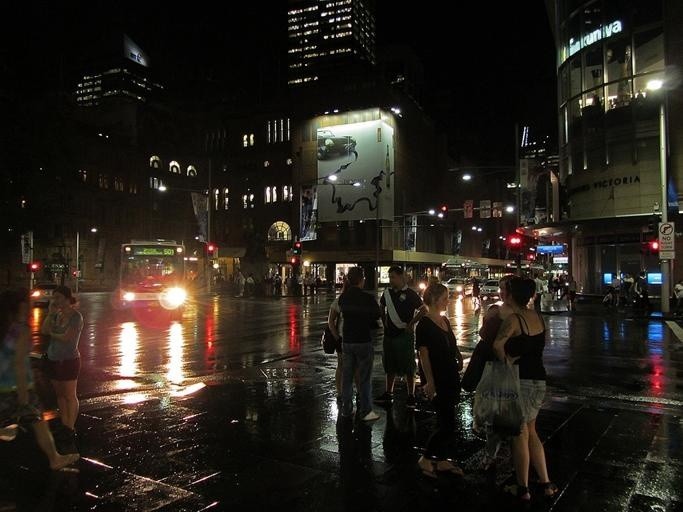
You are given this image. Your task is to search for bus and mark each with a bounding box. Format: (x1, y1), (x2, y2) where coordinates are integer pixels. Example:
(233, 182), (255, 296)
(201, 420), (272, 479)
(119, 239), (185, 319)
(440, 256), (489, 280)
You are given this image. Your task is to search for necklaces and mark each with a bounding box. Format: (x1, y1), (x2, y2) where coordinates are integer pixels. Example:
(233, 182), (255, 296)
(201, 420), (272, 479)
(435, 317), (445, 328)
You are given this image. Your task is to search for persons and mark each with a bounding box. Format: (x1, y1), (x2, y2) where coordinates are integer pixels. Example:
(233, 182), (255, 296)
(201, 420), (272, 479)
(217, 268), (321, 298)
(320, 279), (359, 416)
(40, 285), (84, 440)
(371, 266), (428, 407)
(534, 272), (545, 313)
(337, 267), (380, 420)
(548, 270), (651, 309)
(470, 278), (562, 502)
(459, 275), (517, 478)
(415, 276), (466, 484)
(0, 291), (81, 470)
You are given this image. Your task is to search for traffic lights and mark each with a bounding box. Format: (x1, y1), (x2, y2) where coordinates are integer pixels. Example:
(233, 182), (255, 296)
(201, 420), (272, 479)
(73, 271), (76, 278)
(208, 245), (213, 256)
(441, 205), (448, 215)
(32, 265), (38, 272)
(650, 239), (659, 254)
(290, 242), (302, 266)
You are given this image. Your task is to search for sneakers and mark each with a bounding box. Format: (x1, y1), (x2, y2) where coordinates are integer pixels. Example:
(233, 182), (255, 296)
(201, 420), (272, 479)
(359, 410), (380, 422)
(373, 392), (395, 404)
(336, 393), (358, 418)
(405, 397), (419, 409)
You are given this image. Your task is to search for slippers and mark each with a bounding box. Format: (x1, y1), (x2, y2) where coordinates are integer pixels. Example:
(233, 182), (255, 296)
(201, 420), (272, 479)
(415, 458), (439, 483)
(435, 462), (464, 478)
(48, 427), (81, 473)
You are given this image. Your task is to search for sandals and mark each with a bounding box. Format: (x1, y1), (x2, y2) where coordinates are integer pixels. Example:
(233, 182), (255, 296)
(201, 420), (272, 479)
(544, 481), (560, 502)
(502, 483), (535, 504)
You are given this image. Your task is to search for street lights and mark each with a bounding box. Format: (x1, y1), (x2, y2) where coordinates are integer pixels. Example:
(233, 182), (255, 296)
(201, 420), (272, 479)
(299, 175), (362, 236)
(646, 78), (671, 313)
(158, 184), (212, 293)
(75, 228), (99, 291)
(402, 209), (444, 253)
(448, 165), (524, 274)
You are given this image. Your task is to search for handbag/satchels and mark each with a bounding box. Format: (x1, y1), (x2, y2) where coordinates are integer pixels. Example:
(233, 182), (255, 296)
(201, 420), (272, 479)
(461, 340), (496, 392)
(472, 359), (522, 441)
(320, 325), (336, 354)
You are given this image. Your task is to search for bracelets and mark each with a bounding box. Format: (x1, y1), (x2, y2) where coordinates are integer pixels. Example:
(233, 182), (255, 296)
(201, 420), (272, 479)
(47, 312), (56, 319)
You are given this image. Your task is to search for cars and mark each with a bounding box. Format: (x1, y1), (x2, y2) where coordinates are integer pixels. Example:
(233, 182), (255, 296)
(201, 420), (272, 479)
(419, 276), (503, 300)
(317, 129), (357, 160)
(32, 283), (58, 306)
